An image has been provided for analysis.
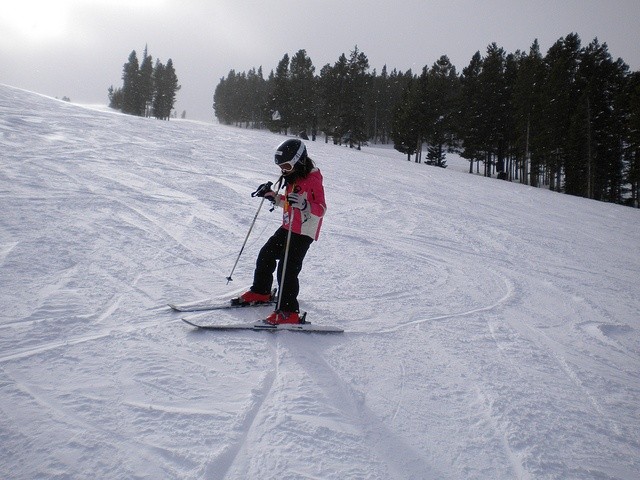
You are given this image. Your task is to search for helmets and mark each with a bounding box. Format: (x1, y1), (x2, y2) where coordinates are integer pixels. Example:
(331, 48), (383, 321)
(274, 138), (308, 182)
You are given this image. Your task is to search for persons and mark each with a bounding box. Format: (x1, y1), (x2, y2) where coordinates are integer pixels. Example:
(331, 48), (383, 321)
(233, 139), (328, 325)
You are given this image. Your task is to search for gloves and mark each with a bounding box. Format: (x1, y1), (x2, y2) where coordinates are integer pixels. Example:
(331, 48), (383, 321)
(286, 193), (307, 210)
(250, 183), (281, 212)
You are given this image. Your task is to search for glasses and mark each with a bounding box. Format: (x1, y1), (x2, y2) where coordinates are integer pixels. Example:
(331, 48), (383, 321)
(278, 139), (305, 172)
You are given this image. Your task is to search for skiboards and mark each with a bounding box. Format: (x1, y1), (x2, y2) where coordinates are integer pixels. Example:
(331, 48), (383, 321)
(178, 317), (346, 338)
(165, 290), (277, 314)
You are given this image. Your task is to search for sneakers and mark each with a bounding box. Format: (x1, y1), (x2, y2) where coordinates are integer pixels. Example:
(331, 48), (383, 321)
(265, 310), (301, 324)
(240, 291), (271, 303)
